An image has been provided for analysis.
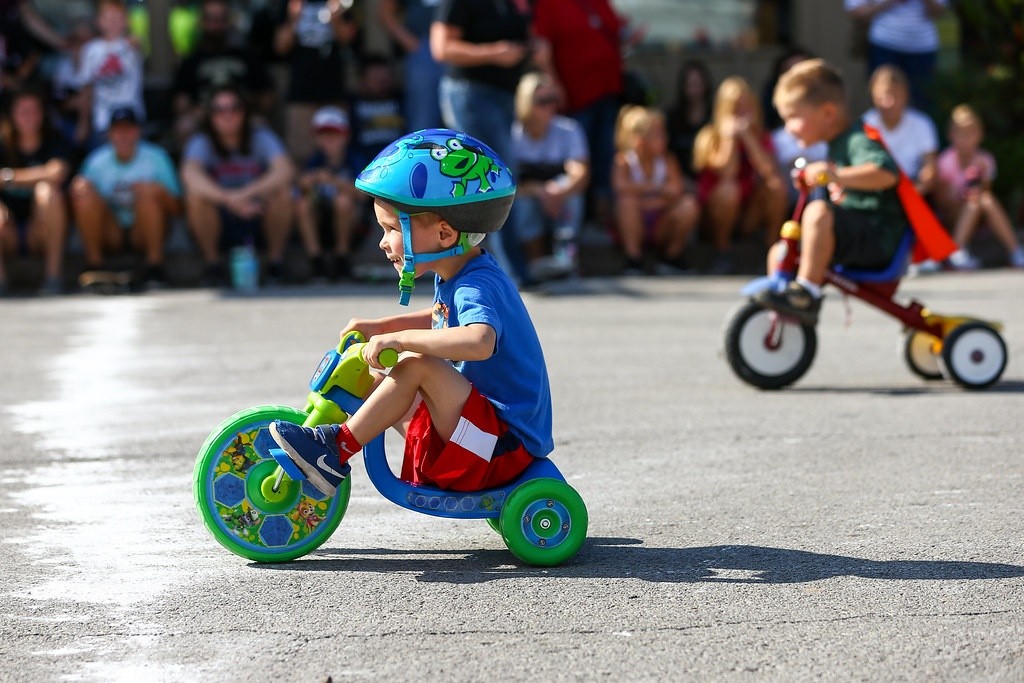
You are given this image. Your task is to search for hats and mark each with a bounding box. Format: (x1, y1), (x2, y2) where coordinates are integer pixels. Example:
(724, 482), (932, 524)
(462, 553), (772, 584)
(107, 109), (138, 128)
(205, 67), (244, 102)
(308, 107), (351, 131)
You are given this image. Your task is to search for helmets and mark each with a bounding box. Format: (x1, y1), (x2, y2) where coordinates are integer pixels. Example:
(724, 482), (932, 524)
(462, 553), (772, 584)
(354, 128), (517, 233)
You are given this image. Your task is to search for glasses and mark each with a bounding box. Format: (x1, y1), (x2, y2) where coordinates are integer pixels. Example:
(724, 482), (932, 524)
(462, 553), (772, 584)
(528, 92), (557, 108)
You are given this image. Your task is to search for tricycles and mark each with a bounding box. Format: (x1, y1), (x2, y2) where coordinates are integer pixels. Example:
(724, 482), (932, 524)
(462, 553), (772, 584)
(723, 158), (1009, 390)
(193, 330), (588, 567)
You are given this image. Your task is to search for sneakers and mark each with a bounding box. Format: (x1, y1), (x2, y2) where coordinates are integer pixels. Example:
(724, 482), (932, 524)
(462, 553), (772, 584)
(269, 420), (351, 497)
(756, 280), (825, 327)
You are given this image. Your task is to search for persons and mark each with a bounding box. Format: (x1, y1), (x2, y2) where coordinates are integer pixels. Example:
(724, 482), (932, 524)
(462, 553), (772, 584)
(268, 128), (555, 497)
(750, 58), (909, 327)
(0, 0), (1023, 294)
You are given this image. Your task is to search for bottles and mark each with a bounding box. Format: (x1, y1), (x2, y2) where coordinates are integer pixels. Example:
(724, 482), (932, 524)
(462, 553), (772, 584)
(231, 245), (259, 296)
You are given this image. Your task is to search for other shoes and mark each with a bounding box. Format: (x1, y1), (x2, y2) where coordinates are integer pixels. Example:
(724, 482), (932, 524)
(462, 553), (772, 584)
(546, 243), (578, 272)
(523, 268), (566, 282)
